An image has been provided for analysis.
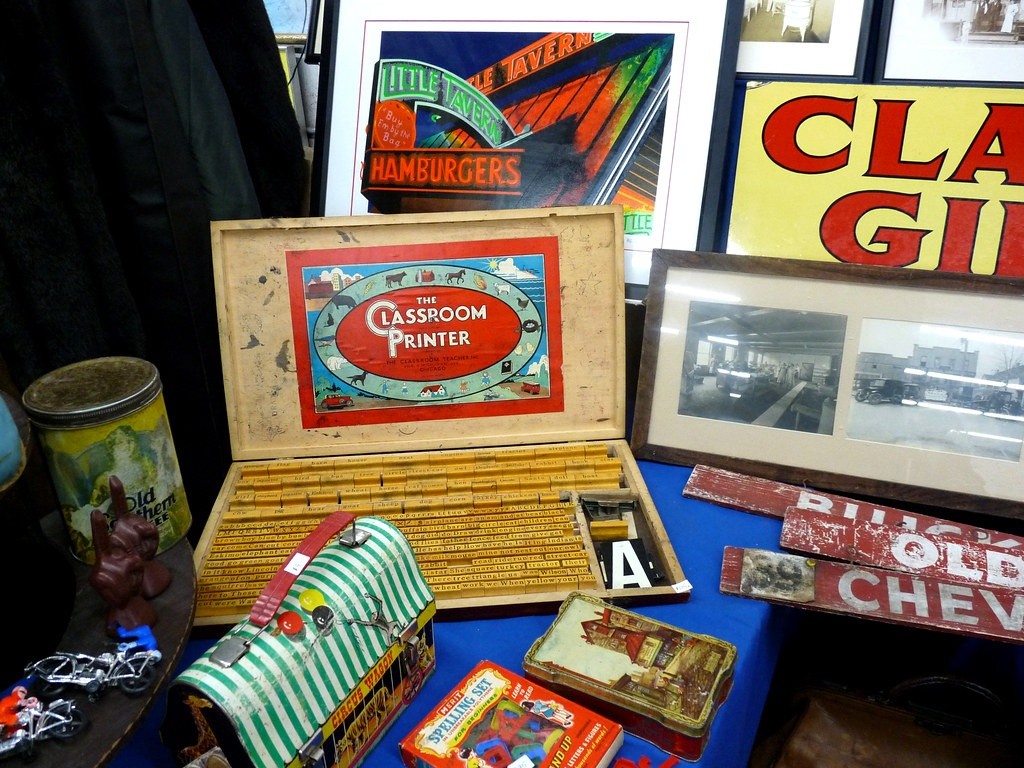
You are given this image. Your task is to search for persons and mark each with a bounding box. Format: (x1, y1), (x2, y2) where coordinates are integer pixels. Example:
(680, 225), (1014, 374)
(972, 0), (1019, 33)
(777, 362), (799, 389)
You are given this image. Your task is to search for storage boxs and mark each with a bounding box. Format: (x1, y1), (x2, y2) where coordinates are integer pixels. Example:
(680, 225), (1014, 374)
(190, 202), (691, 631)
(522, 592), (737, 763)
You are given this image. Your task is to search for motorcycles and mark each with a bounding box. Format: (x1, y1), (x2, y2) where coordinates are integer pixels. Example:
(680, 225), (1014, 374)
(31, 650), (163, 699)
(0, 697), (90, 756)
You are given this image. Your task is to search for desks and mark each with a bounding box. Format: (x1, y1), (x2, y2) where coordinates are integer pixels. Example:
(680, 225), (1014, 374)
(105, 456), (778, 768)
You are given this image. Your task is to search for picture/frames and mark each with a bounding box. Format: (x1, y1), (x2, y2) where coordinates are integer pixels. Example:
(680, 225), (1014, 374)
(695, 0), (1024, 279)
(627, 245), (1024, 523)
(265, 1), (325, 146)
(307, 0), (743, 305)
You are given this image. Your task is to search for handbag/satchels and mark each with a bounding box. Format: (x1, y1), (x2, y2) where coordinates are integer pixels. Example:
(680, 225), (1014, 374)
(160, 509), (437, 768)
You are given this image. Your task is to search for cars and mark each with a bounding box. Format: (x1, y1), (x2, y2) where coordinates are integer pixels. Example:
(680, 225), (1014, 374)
(854, 377), (905, 405)
(977, 390), (1021, 415)
(904, 383), (921, 400)
(679, 350), (698, 399)
(715, 361), (776, 396)
